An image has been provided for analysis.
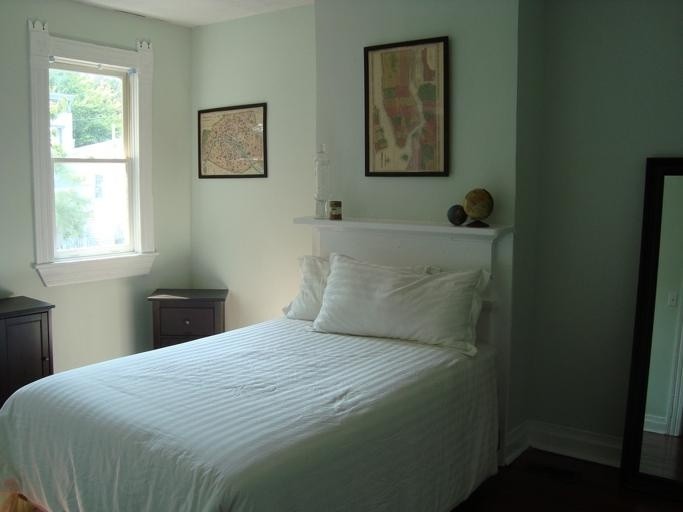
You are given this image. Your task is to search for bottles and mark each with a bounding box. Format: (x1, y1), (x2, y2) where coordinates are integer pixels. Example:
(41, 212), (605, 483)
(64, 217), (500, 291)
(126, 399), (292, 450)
(313, 142), (341, 220)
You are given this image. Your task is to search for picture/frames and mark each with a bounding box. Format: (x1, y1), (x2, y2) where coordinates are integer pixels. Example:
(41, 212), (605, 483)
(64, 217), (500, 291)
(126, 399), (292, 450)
(197, 103), (267, 178)
(363, 37), (450, 177)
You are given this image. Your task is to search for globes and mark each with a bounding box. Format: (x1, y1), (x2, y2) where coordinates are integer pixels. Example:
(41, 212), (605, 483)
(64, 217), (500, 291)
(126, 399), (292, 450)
(463, 188), (494, 228)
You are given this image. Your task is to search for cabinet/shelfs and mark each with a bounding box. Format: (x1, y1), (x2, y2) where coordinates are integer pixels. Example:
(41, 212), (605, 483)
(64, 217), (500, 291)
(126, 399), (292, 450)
(0, 296), (55, 407)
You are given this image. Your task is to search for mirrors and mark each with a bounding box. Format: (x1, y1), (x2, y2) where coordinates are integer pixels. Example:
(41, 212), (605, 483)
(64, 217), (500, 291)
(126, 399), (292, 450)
(621, 157), (683, 511)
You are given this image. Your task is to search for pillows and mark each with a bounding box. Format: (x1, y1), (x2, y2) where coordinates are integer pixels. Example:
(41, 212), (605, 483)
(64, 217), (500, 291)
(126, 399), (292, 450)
(306, 251), (491, 357)
(282, 252), (443, 322)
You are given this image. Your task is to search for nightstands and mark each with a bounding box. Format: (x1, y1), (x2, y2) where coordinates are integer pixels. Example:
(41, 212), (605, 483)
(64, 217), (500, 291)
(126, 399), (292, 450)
(150, 289), (228, 347)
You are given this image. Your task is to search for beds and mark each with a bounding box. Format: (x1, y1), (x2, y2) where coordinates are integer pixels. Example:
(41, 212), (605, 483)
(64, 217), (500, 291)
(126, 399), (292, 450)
(0, 217), (512, 512)
